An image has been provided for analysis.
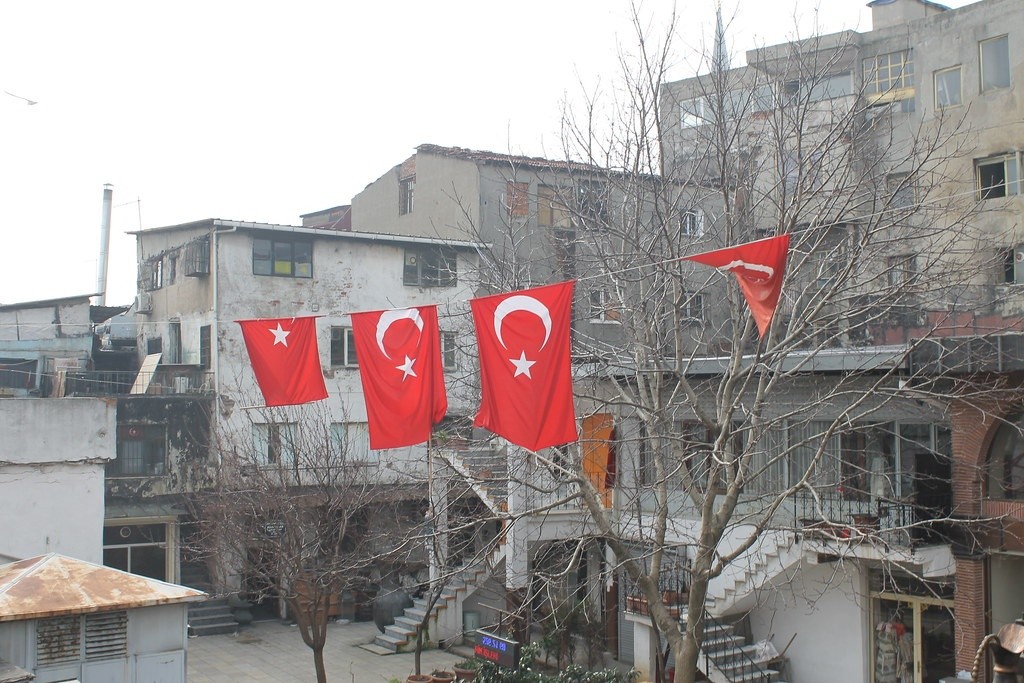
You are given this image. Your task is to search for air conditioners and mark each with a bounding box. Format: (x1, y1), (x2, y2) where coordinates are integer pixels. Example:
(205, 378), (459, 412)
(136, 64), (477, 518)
(134, 293), (150, 313)
(171, 376), (188, 393)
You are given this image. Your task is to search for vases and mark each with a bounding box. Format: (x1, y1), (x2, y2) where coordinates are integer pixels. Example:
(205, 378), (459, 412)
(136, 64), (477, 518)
(408, 674), (433, 683)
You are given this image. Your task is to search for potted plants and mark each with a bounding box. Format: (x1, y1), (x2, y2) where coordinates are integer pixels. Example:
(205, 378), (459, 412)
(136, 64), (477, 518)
(430, 665), (455, 683)
(453, 658), (479, 680)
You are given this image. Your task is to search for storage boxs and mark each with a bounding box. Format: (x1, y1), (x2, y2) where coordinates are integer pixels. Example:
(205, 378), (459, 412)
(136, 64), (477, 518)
(150, 383), (161, 394)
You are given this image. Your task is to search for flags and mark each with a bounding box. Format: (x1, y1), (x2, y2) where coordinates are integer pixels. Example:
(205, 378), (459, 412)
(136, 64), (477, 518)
(685, 233), (790, 340)
(238, 315), (329, 407)
(469, 279), (579, 452)
(351, 305), (448, 450)
